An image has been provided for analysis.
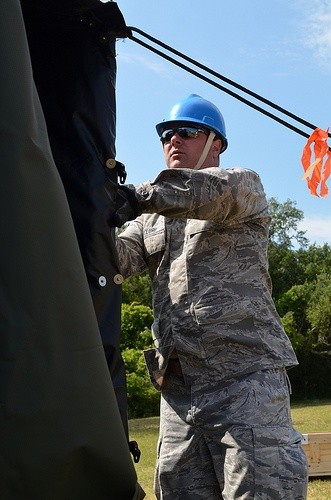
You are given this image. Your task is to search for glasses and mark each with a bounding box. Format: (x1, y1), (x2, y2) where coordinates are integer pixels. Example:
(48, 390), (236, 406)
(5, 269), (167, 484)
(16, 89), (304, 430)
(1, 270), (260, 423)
(160, 128), (209, 142)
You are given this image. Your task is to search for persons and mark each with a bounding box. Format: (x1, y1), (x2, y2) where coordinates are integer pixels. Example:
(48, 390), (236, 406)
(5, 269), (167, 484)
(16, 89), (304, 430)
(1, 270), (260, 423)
(113, 94), (309, 500)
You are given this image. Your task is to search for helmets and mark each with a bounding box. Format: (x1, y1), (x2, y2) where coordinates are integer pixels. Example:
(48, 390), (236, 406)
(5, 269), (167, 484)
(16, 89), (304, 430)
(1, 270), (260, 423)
(154, 93), (228, 153)
